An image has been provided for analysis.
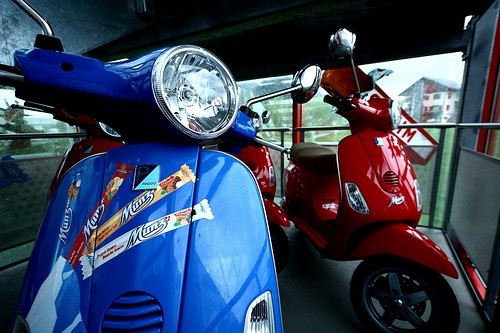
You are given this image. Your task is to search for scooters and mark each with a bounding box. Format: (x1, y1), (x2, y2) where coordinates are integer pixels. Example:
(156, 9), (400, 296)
(0, 1), (461, 333)
